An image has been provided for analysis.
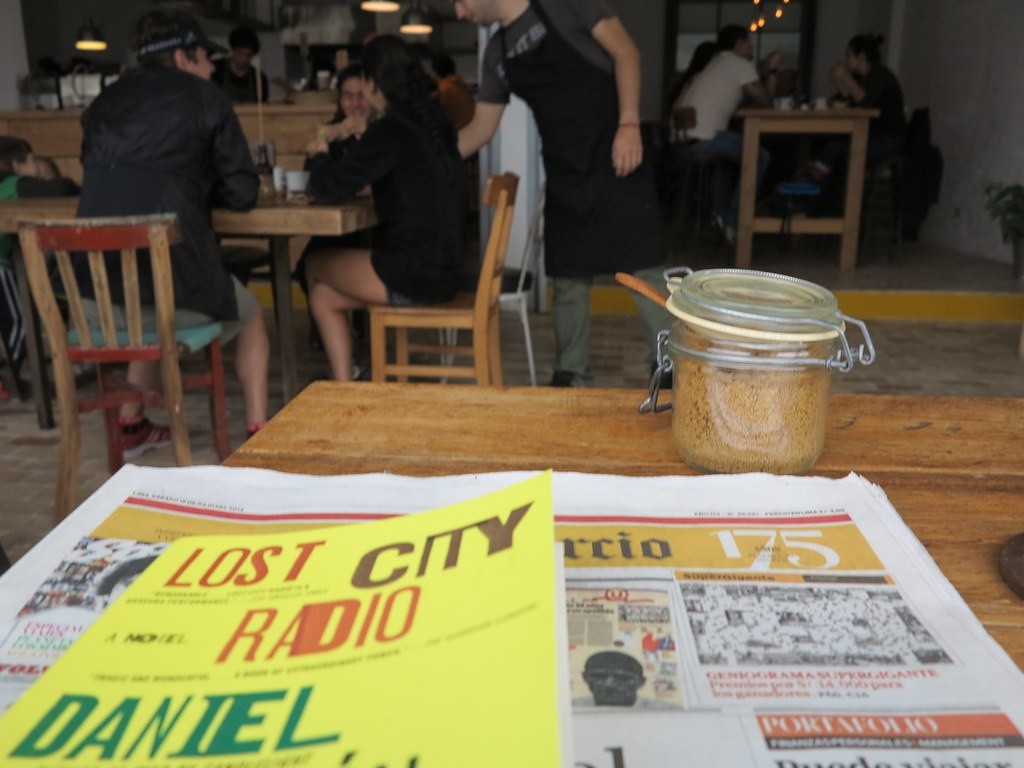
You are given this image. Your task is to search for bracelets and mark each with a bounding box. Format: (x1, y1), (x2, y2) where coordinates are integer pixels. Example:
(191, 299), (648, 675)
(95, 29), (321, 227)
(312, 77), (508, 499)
(619, 123), (640, 129)
(768, 68), (776, 73)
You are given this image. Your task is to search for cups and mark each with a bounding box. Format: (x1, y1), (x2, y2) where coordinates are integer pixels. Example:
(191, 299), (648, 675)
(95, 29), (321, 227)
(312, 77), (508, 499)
(285, 170), (312, 192)
(272, 165), (299, 193)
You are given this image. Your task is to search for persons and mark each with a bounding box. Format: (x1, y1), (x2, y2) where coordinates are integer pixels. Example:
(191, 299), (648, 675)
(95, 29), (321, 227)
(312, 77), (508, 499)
(210, 25), (268, 104)
(806, 33), (907, 252)
(292, 64), (378, 350)
(72, 9), (271, 462)
(304, 31), (470, 381)
(0, 134), (81, 402)
(438, 65), (469, 100)
(456, 0), (690, 389)
(661, 25), (781, 247)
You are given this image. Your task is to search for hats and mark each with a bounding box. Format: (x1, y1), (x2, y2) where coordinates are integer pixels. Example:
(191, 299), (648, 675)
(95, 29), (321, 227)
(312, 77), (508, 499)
(134, 21), (230, 58)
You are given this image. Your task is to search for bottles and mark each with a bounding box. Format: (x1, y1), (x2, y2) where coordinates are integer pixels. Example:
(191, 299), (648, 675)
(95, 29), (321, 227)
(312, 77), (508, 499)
(638, 266), (874, 477)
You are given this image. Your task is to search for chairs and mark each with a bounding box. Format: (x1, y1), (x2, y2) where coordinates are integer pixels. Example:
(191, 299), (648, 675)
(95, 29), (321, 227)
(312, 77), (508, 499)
(622, 103), (946, 259)
(14, 210), (234, 528)
(367, 172), (521, 394)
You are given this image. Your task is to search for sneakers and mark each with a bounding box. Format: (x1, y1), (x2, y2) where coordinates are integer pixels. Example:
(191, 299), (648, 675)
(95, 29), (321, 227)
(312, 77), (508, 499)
(118, 424), (175, 459)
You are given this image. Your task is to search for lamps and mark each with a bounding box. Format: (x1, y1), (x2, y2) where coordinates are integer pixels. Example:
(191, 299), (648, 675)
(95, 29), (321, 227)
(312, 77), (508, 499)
(360, 0), (434, 36)
(75, 16), (107, 51)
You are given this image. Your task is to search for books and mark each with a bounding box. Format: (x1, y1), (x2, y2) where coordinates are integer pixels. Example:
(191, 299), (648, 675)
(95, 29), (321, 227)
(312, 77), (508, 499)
(0, 469), (575, 768)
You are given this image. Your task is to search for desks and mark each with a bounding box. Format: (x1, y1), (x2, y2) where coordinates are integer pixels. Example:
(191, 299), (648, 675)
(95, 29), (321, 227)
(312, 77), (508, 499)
(734, 107), (880, 273)
(0, 381), (1024, 768)
(0, 194), (382, 433)
